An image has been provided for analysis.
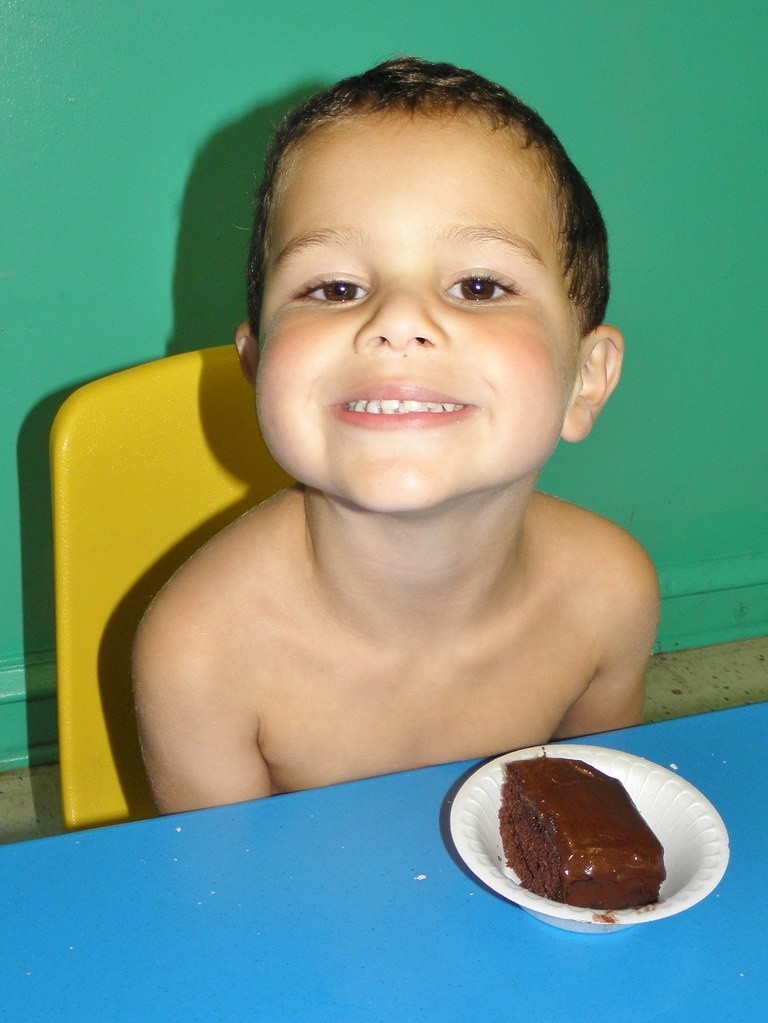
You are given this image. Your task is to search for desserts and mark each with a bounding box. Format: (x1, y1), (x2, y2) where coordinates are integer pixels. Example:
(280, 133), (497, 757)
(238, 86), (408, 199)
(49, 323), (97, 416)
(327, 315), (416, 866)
(498, 758), (667, 909)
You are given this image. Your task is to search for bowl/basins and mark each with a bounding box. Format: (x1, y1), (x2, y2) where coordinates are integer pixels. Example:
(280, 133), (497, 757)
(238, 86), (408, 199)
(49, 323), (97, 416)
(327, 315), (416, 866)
(449, 743), (731, 935)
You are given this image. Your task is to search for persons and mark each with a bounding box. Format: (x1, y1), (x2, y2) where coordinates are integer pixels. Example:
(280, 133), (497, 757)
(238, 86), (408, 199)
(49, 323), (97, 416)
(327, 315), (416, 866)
(133, 57), (653, 815)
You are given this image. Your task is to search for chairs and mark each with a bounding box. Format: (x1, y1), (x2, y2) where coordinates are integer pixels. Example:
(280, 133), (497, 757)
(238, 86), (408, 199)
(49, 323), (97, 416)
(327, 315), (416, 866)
(47, 341), (301, 833)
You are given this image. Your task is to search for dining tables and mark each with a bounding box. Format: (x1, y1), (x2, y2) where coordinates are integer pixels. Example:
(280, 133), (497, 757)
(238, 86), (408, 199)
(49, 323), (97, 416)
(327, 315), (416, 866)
(1, 699), (767, 1022)
